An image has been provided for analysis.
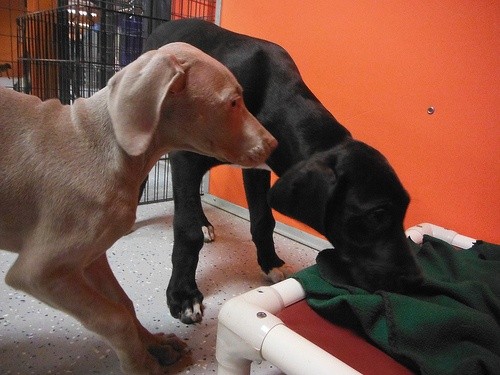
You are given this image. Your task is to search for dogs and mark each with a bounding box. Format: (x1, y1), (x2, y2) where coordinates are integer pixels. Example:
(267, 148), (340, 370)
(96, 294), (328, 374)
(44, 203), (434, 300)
(137, 18), (426, 325)
(0, 41), (279, 375)
